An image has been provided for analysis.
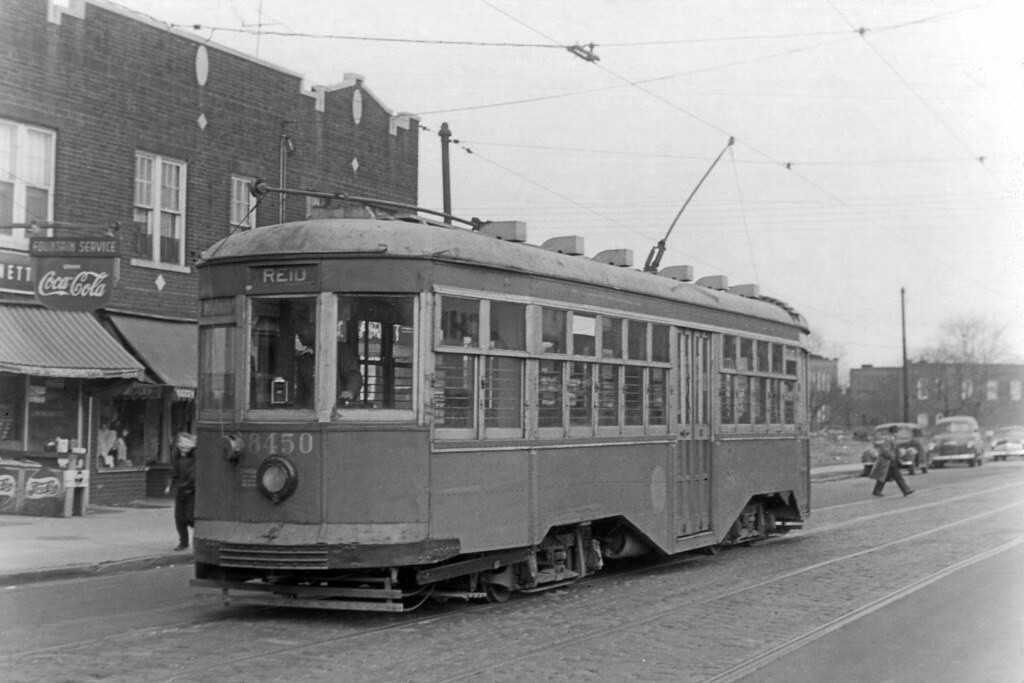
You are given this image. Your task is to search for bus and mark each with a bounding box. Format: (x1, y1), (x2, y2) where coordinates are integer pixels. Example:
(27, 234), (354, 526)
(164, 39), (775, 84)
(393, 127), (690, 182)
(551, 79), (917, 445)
(191, 219), (818, 615)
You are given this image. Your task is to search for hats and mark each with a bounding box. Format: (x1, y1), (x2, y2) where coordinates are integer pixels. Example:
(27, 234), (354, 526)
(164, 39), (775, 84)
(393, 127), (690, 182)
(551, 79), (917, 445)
(889, 426), (902, 433)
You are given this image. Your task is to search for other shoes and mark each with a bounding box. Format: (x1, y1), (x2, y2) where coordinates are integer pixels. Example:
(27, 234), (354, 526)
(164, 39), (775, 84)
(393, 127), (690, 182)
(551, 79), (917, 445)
(174, 543), (188, 551)
(872, 492), (884, 496)
(904, 489), (916, 496)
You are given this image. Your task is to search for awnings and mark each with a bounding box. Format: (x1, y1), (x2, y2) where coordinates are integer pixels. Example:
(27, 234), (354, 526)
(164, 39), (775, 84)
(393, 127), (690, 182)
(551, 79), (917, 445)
(90, 311), (199, 404)
(0, 306), (145, 380)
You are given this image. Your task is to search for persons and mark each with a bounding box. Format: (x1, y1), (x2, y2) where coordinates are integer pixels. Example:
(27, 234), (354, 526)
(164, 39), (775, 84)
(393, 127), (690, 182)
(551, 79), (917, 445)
(165, 432), (197, 551)
(870, 426), (915, 495)
(294, 317), (363, 400)
(98, 421), (129, 466)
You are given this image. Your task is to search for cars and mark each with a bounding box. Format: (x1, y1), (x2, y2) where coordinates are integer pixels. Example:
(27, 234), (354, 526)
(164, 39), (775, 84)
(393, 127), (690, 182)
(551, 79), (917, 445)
(984, 422), (1023, 471)
(922, 414), (985, 470)
(861, 423), (926, 477)
